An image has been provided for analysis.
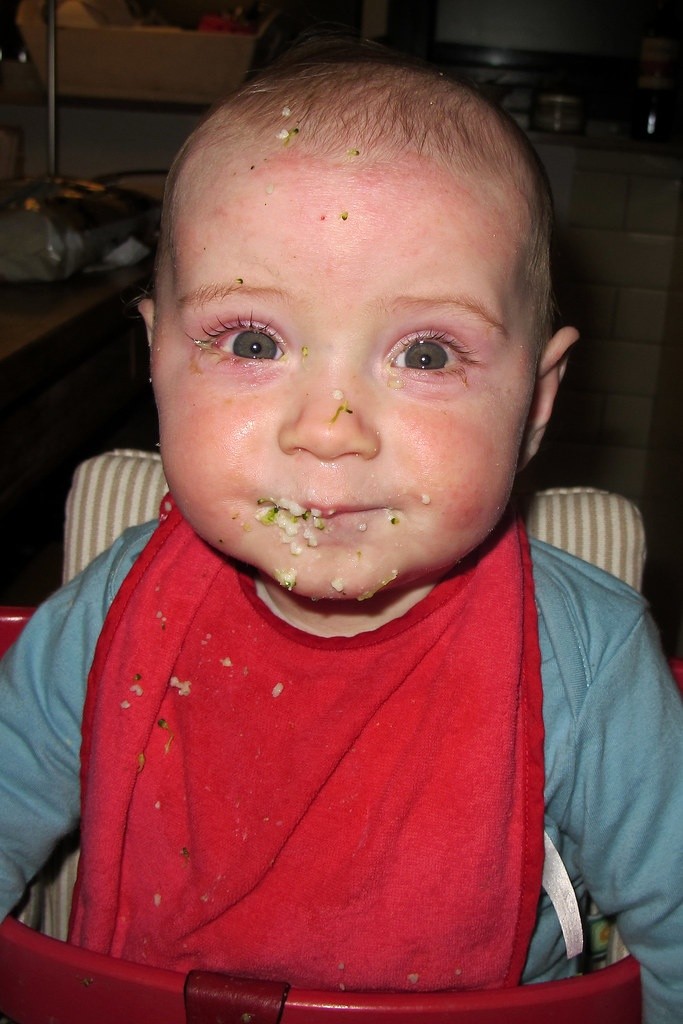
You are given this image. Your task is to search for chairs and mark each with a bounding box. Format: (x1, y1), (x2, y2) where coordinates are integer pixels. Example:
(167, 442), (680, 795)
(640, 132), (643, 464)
(0, 446), (683, 1024)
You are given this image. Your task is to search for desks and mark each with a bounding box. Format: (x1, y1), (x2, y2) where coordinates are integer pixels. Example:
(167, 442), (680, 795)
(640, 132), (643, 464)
(1, 263), (153, 595)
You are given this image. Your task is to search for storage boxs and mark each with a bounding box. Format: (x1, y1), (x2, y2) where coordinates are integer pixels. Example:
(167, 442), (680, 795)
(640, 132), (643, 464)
(16, 0), (283, 105)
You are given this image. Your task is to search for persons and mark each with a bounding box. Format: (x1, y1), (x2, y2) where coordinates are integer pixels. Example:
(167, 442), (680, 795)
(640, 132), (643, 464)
(0, 61), (682, 1024)
